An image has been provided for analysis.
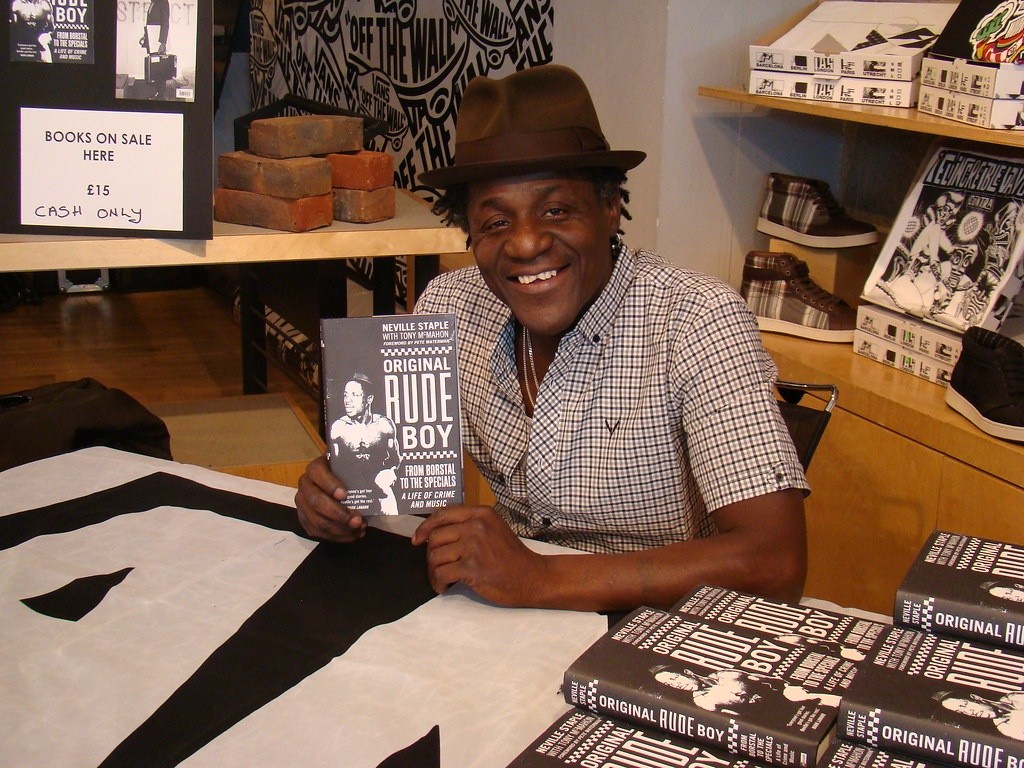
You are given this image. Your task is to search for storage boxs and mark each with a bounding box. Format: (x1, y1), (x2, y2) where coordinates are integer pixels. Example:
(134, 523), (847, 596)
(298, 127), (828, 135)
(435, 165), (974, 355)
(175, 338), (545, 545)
(748, 0), (1024, 131)
(853, 146), (1024, 388)
(769, 201), (895, 313)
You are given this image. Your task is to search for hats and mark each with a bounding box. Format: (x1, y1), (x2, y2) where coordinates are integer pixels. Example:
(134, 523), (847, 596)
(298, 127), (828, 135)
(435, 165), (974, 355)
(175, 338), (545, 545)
(416, 65), (646, 190)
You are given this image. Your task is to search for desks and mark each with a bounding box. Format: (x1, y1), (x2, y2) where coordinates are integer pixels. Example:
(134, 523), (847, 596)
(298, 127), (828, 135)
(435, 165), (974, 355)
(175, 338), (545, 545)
(0, 443), (888, 768)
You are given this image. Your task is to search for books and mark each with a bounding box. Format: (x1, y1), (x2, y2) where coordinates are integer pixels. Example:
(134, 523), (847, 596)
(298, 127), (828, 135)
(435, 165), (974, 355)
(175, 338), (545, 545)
(504, 529), (1024, 768)
(320, 313), (466, 516)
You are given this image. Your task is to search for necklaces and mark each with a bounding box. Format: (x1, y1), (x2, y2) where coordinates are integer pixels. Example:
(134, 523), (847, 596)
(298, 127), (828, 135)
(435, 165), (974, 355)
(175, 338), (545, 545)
(523, 326), (539, 408)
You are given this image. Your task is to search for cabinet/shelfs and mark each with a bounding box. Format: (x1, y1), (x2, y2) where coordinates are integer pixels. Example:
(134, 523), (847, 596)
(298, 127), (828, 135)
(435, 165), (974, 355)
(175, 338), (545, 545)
(694, 86), (1024, 616)
(0, 0), (470, 490)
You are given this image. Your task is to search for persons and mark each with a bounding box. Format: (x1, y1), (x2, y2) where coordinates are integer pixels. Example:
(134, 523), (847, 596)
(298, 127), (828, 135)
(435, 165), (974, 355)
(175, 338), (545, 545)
(138, 0), (170, 100)
(9, 0), (56, 63)
(295, 64), (812, 612)
(329, 372), (399, 516)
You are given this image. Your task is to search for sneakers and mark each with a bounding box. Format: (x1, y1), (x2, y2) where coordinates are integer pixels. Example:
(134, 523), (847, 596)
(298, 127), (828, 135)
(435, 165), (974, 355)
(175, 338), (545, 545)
(741, 252), (857, 342)
(944, 326), (1024, 442)
(757, 172), (878, 248)
